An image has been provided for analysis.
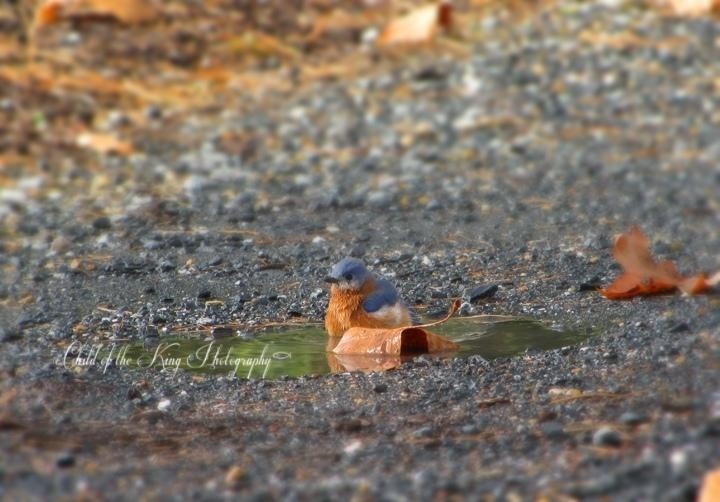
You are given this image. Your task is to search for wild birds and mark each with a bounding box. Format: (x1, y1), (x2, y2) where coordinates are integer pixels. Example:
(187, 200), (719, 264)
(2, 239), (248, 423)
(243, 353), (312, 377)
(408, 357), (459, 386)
(322, 256), (421, 338)
